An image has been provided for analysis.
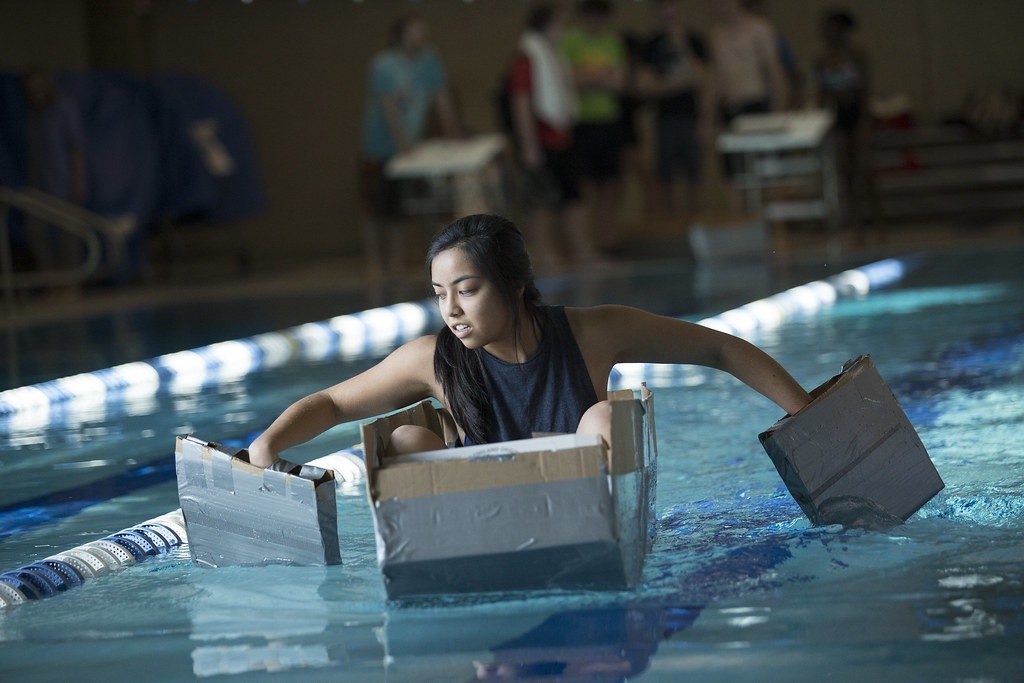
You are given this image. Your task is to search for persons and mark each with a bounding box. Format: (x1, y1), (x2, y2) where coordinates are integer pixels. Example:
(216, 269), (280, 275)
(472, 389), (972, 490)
(247, 214), (813, 470)
(357, 0), (877, 290)
